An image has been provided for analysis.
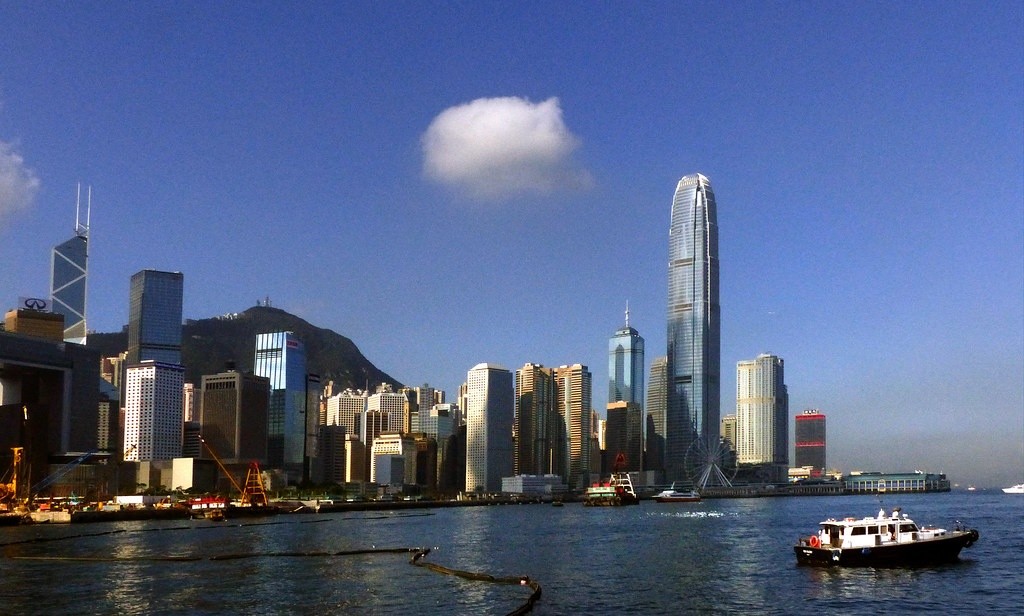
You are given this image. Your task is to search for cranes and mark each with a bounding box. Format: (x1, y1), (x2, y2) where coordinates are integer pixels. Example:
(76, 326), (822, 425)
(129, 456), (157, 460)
(68, 444), (138, 515)
(29, 445), (99, 506)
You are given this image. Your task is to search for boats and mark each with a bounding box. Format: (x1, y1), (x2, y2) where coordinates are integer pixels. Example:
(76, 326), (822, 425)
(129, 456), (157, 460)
(794, 493), (980, 568)
(579, 472), (640, 507)
(650, 480), (701, 502)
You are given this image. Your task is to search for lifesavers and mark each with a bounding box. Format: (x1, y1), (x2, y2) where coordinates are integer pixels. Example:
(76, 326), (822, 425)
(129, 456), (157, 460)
(810, 535), (818, 547)
(963, 528), (979, 548)
